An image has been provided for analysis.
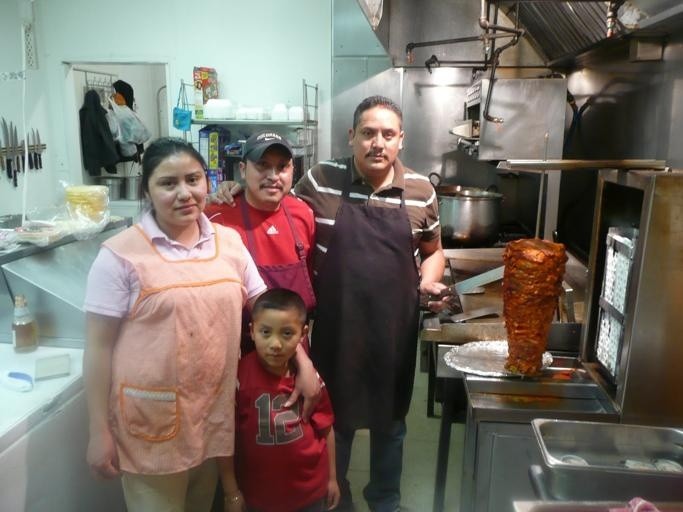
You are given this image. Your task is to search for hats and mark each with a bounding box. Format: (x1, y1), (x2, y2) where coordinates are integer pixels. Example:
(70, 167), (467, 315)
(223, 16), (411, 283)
(243, 128), (294, 163)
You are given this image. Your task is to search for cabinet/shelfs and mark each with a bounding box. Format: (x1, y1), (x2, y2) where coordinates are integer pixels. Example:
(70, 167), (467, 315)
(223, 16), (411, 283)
(180, 77), (320, 195)
(1, 215), (154, 512)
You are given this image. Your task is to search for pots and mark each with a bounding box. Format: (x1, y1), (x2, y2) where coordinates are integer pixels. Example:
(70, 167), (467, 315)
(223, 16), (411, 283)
(98, 176), (143, 201)
(429, 172), (501, 246)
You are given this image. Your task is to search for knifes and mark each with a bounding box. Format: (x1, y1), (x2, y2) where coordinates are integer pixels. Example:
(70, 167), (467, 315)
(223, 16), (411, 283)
(420, 265), (504, 304)
(0, 116), (42, 187)
(437, 308), (496, 323)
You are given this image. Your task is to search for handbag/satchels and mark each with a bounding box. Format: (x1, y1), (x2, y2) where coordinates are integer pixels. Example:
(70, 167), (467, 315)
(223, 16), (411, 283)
(172, 83), (192, 131)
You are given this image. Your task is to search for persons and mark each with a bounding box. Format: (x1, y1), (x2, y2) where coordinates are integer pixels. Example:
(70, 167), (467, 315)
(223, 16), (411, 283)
(202, 129), (316, 367)
(216, 286), (341, 512)
(82, 136), (322, 512)
(209, 95), (458, 512)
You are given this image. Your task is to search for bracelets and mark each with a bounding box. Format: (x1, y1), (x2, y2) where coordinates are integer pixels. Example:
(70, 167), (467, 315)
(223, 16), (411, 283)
(224, 491), (244, 507)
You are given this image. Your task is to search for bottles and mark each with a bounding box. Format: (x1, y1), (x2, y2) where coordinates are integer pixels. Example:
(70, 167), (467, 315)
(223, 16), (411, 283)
(11, 294), (38, 354)
